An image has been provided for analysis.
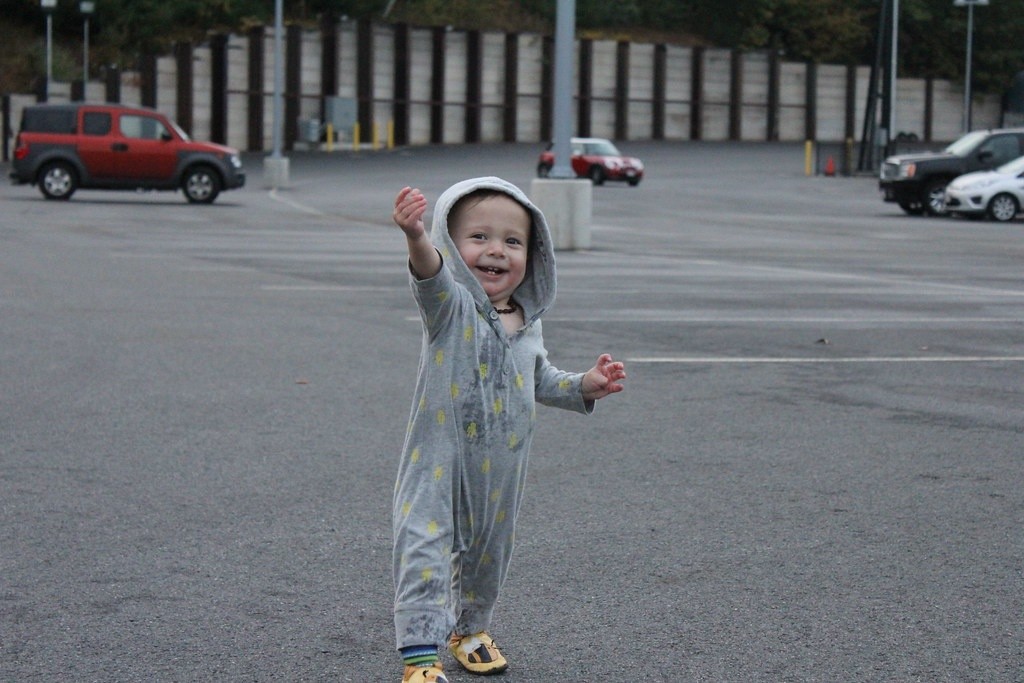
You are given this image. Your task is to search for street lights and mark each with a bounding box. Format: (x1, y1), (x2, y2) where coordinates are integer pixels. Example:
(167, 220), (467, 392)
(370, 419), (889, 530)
(42, 0), (57, 103)
(80, 0), (96, 102)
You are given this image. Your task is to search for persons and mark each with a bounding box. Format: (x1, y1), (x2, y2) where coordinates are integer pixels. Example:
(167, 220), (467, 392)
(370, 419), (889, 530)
(392, 176), (626, 683)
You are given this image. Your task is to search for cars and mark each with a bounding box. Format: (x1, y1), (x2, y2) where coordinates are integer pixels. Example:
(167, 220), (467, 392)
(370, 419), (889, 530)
(944, 152), (1024, 223)
(536, 137), (645, 186)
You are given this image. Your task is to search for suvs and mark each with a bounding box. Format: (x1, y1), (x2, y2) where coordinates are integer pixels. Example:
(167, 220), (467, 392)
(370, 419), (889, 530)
(877, 125), (1024, 217)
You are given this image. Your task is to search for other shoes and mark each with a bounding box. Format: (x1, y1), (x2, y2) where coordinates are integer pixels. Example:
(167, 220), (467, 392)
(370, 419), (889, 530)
(400, 660), (448, 683)
(449, 630), (508, 674)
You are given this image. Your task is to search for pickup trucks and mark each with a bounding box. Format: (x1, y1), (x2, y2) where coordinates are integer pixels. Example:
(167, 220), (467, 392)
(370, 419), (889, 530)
(8, 102), (245, 205)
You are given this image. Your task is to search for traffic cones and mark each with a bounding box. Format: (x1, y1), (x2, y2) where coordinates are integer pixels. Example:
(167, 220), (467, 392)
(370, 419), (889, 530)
(824, 155), (836, 177)
